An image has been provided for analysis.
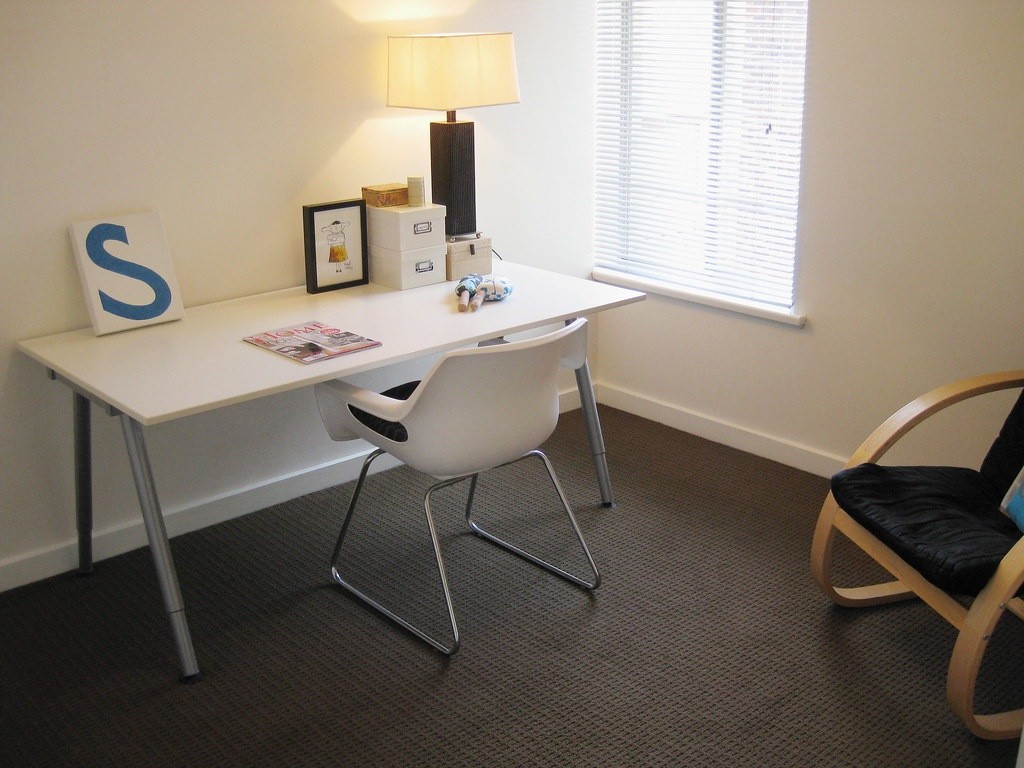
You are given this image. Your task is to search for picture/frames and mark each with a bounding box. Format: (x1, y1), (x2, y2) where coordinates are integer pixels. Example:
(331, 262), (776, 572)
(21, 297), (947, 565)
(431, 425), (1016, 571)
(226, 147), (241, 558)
(302, 198), (370, 295)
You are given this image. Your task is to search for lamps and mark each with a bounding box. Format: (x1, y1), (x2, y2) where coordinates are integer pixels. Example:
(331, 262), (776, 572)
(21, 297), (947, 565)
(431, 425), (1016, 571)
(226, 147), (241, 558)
(386, 31), (521, 235)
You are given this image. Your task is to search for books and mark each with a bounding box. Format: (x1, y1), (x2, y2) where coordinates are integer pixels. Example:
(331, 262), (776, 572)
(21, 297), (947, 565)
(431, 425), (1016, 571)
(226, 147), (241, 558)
(241, 320), (383, 365)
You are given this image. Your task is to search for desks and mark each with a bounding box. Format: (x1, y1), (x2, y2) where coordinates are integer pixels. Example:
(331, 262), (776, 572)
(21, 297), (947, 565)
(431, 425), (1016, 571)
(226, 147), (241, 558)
(14, 258), (647, 685)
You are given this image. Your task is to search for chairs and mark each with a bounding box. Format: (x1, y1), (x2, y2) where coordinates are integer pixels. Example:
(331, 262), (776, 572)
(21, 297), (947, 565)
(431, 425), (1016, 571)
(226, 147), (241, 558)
(312, 317), (602, 657)
(809, 371), (1024, 740)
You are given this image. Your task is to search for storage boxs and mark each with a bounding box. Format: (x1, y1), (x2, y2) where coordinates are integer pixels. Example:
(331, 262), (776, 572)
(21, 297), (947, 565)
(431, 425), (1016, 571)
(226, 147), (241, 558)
(367, 243), (447, 291)
(446, 236), (492, 282)
(361, 182), (407, 208)
(366, 204), (447, 252)
